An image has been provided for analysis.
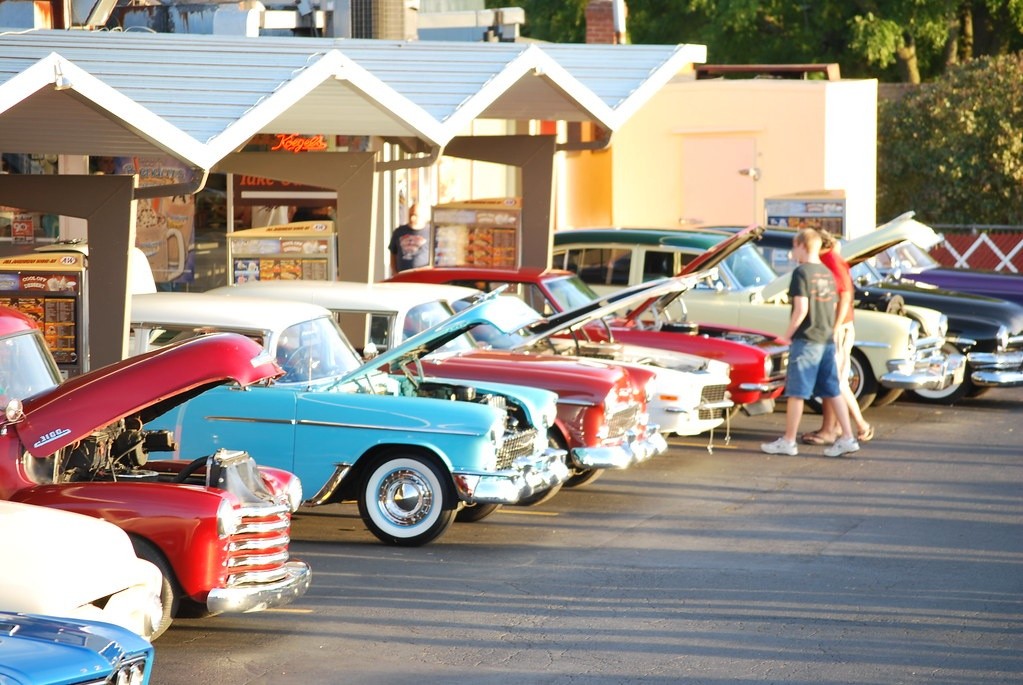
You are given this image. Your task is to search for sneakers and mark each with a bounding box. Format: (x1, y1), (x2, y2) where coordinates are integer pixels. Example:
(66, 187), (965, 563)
(761, 437), (799, 456)
(823, 437), (860, 457)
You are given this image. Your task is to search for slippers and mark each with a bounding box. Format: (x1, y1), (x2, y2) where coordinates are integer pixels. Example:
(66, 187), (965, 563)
(857, 427), (875, 442)
(801, 432), (835, 445)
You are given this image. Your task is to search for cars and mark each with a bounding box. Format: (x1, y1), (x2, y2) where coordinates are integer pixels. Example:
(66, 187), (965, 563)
(0, 500), (172, 646)
(2, 308), (314, 621)
(0, 610), (154, 685)
(122, 208), (1020, 550)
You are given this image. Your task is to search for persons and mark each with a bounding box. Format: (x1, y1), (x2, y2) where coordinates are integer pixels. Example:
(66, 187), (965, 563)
(242, 204), (333, 228)
(388, 202), (431, 277)
(760, 226), (875, 456)
